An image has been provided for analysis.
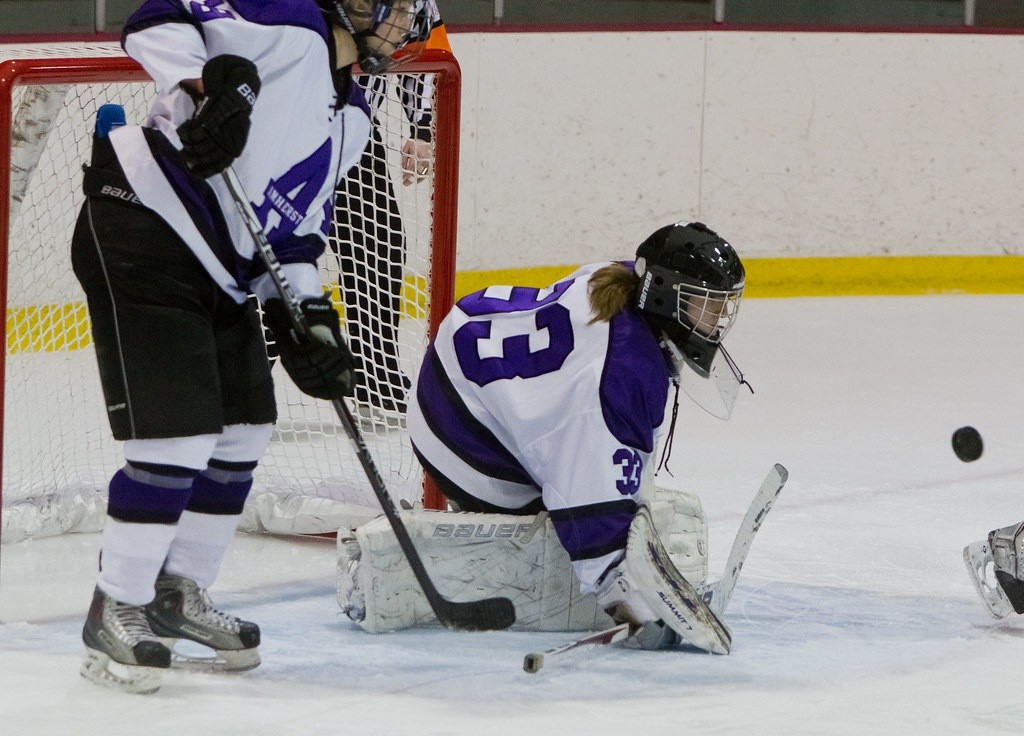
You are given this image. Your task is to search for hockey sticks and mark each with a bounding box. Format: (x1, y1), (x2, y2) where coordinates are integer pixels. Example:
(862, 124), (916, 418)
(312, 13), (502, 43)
(192, 99), (517, 631)
(524, 462), (790, 675)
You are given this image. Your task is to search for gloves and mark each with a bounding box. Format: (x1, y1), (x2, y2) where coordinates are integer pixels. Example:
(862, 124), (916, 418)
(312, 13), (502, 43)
(263, 295), (356, 402)
(593, 552), (677, 653)
(179, 54), (262, 191)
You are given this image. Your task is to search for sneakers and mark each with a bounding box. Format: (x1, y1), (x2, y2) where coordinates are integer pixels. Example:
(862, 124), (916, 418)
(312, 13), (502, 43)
(76, 582), (171, 696)
(964, 522), (1024, 630)
(144, 568), (260, 673)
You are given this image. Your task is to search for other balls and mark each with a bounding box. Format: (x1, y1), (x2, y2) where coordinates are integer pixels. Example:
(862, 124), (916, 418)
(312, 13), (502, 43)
(952, 426), (984, 463)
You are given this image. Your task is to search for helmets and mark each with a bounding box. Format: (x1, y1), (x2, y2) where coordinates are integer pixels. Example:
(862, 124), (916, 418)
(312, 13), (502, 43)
(323, 0), (415, 76)
(632, 221), (747, 379)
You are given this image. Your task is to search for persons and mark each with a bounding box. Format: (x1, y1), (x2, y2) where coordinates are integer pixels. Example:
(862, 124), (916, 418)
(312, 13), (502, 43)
(70, 0), (455, 694)
(405, 221), (744, 632)
